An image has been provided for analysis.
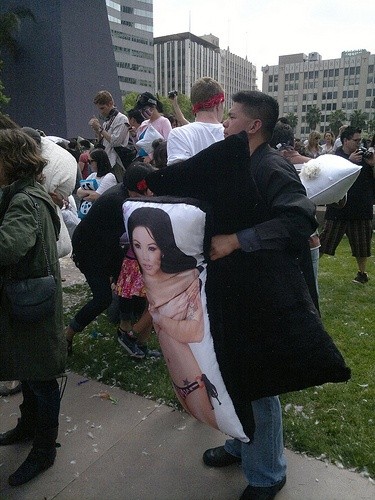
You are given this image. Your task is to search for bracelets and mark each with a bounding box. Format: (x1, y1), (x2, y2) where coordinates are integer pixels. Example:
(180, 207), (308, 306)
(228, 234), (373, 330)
(98, 127), (104, 133)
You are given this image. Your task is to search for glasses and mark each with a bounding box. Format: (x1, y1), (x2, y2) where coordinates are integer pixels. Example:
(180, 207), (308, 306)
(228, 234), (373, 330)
(86, 159), (95, 165)
(349, 137), (362, 143)
(139, 104), (151, 112)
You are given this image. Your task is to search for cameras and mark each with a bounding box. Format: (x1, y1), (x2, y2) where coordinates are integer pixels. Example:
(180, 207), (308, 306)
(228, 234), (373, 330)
(356, 146), (372, 158)
(168, 90), (177, 99)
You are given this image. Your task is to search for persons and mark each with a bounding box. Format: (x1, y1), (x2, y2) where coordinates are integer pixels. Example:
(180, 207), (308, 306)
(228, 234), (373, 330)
(271, 123), (348, 279)
(0, 129), (66, 486)
(319, 126), (375, 284)
(166, 77), (227, 165)
(66, 139), (91, 180)
(125, 207), (218, 432)
(203, 90), (319, 500)
(129, 90), (189, 164)
(77, 150), (116, 202)
(66, 163), (161, 358)
(89, 91), (131, 182)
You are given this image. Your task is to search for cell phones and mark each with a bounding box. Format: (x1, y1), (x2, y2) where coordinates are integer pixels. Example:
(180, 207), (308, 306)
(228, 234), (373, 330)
(124, 122), (133, 130)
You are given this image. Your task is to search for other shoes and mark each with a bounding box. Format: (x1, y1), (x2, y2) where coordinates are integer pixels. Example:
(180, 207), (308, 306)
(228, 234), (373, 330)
(117, 327), (127, 338)
(8, 450), (54, 486)
(0, 418), (34, 446)
(0, 379), (21, 396)
(203, 446), (241, 467)
(239, 473), (287, 500)
(64, 335), (73, 351)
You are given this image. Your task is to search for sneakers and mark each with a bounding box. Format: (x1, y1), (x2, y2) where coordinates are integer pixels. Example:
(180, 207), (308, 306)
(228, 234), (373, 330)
(117, 334), (145, 358)
(137, 344), (161, 357)
(352, 270), (370, 285)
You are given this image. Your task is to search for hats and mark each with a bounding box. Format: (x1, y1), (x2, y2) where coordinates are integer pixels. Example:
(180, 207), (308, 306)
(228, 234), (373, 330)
(21, 127), (41, 143)
(134, 93), (157, 109)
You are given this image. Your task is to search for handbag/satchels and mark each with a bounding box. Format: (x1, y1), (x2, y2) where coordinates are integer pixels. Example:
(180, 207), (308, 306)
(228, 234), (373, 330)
(0, 275), (57, 323)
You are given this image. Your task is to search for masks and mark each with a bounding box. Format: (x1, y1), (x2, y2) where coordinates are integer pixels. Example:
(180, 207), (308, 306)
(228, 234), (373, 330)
(141, 108), (153, 119)
